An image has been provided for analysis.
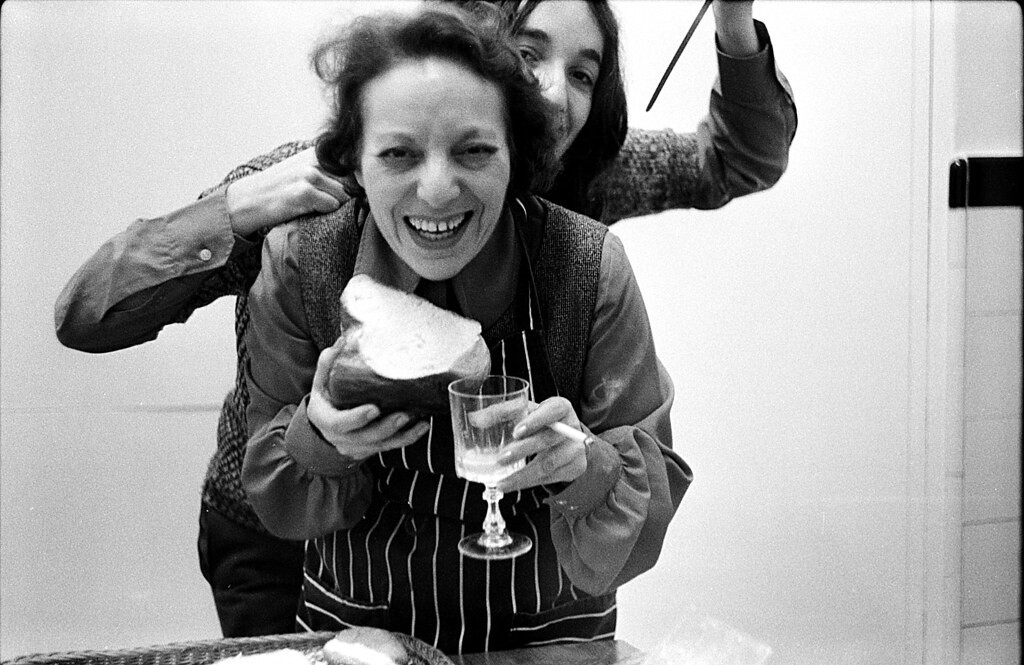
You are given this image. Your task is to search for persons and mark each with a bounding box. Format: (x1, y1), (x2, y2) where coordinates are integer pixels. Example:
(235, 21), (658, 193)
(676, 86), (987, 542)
(238, 6), (694, 656)
(54, 1), (795, 639)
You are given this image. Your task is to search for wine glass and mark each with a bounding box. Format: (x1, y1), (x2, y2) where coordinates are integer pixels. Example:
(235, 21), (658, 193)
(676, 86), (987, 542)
(442, 377), (537, 561)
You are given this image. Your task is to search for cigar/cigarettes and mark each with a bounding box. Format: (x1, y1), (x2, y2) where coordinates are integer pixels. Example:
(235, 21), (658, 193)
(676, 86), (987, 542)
(545, 422), (594, 446)
